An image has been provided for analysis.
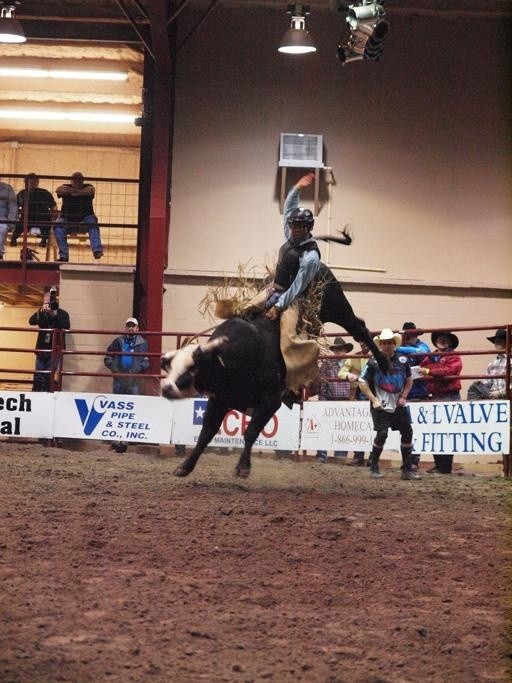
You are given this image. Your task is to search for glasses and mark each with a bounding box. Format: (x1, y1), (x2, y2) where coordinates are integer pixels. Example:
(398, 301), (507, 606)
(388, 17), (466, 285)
(380, 340), (395, 345)
(126, 323), (136, 328)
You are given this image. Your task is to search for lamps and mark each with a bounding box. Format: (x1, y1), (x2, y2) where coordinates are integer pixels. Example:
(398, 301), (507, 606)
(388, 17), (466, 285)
(0, 0), (26, 43)
(277, 4), (317, 54)
(336, 0), (390, 66)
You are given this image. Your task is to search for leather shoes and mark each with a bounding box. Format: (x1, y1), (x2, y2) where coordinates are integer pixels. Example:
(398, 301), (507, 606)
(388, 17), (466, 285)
(57, 258), (69, 263)
(94, 251), (103, 259)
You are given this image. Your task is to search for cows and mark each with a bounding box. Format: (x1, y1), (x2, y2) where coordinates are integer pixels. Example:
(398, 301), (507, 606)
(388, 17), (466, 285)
(159, 223), (396, 480)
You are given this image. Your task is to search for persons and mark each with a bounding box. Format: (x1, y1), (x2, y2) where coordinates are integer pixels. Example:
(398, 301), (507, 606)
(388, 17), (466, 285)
(479, 329), (512, 399)
(359, 329), (422, 480)
(54, 171), (104, 261)
(230, 172), (322, 410)
(394, 321), (430, 471)
(103, 318), (150, 453)
(0, 181), (17, 260)
(10, 172), (57, 247)
(419, 330), (461, 474)
(30, 292), (70, 391)
(315, 337), (352, 464)
(338, 336), (377, 467)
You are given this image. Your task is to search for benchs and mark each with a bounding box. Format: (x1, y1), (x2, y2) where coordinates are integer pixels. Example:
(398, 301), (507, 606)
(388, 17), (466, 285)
(0, 208), (91, 262)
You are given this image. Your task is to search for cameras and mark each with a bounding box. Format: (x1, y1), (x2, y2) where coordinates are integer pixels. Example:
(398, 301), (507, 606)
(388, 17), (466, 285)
(48, 286), (58, 310)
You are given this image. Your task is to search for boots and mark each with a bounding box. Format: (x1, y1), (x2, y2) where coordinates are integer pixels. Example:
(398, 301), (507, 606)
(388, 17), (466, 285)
(367, 464), (385, 479)
(400, 465), (422, 481)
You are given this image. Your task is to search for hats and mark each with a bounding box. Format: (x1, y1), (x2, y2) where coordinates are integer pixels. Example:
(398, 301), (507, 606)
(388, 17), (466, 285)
(486, 328), (507, 345)
(329, 338), (353, 353)
(399, 323), (423, 336)
(430, 330), (459, 350)
(124, 318), (139, 326)
(373, 328), (403, 350)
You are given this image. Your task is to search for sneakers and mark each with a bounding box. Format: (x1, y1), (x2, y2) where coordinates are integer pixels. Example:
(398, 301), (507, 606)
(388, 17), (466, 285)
(39, 240), (47, 248)
(316, 454), (365, 468)
(10, 239), (17, 246)
(427, 466), (451, 474)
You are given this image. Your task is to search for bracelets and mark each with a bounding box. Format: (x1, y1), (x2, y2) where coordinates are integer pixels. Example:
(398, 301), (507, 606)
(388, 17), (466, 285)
(423, 367), (429, 375)
(399, 393), (408, 399)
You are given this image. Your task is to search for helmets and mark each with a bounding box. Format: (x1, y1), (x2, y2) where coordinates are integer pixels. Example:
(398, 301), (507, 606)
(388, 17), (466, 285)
(283, 207), (316, 246)
